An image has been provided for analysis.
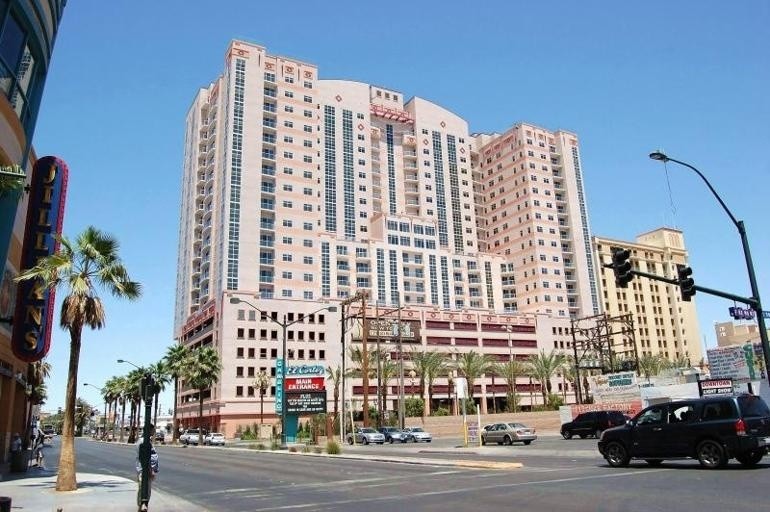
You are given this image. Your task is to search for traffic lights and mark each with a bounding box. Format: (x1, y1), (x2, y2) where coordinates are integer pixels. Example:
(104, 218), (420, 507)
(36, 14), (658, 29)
(610, 244), (635, 288)
(675, 263), (697, 303)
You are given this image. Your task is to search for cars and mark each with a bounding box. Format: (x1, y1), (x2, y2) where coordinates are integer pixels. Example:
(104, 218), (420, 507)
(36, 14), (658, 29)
(400, 426), (433, 443)
(156, 429), (166, 442)
(481, 423), (539, 447)
(480, 423), (494, 434)
(103, 429), (117, 441)
(377, 427), (408, 444)
(202, 432), (227, 446)
(53, 431), (58, 436)
(622, 412), (631, 424)
(345, 427), (386, 446)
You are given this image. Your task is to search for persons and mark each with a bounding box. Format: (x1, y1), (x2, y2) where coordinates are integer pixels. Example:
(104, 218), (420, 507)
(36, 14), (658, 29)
(8, 432), (23, 465)
(134, 423), (163, 511)
(32, 428), (47, 466)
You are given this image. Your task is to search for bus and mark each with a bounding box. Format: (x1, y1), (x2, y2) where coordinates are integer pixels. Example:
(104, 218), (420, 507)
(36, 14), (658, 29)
(43, 423), (54, 432)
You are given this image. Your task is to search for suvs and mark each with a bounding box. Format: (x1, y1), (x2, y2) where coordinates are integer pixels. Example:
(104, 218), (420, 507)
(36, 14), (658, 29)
(597, 393), (770, 468)
(560, 409), (625, 441)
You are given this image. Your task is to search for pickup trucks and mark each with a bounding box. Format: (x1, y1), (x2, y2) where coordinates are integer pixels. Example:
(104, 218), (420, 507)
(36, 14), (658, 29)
(179, 428), (207, 446)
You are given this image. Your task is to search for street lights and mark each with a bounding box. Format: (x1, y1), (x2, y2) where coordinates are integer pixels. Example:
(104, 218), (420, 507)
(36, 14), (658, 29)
(499, 325), (517, 414)
(116, 359), (141, 370)
(646, 149), (770, 383)
(409, 370), (417, 399)
(82, 383), (107, 431)
(448, 370), (455, 403)
(228, 296), (339, 449)
